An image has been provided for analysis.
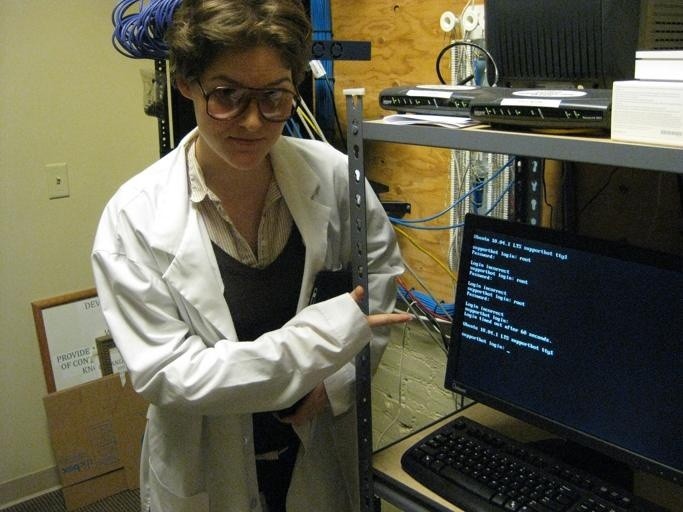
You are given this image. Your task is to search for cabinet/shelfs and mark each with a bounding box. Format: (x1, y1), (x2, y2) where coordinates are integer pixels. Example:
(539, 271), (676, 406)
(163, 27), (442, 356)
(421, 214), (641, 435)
(341, 83), (682, 511)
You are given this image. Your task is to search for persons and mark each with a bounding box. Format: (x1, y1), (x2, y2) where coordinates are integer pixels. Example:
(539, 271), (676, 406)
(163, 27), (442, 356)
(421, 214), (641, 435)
(90, 1), (406, 512)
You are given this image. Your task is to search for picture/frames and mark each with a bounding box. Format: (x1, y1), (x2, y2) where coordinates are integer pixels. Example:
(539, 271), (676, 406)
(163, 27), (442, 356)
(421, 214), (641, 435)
(27, 282), (109, 400)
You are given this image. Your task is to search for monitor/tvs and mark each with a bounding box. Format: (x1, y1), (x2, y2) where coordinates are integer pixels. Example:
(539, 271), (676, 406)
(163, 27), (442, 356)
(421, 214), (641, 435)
(444, 212), (683, 493)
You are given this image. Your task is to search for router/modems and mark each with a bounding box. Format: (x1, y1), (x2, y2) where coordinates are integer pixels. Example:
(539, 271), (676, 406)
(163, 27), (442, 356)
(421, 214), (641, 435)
(469, 88), (612, 130)
(379, 84), (488, 118)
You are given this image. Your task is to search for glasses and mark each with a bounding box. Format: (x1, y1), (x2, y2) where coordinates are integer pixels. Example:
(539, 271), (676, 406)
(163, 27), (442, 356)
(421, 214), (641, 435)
(197, 81), (301, 123)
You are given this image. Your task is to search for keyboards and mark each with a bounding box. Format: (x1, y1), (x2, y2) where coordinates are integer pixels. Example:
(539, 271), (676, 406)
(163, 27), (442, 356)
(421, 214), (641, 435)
(401, 416), (676, 512)
